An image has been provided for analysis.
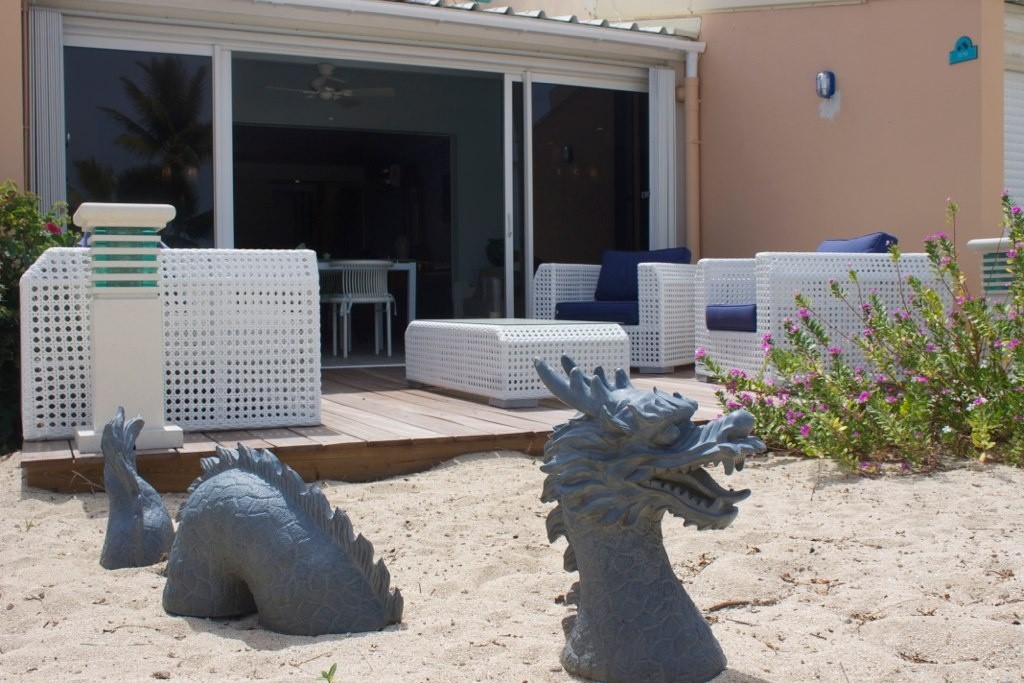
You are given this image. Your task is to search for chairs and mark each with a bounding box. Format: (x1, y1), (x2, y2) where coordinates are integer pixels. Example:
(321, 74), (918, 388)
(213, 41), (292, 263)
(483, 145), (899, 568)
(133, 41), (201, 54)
(19, 249), (322, 440)
(694, 235), (955, 393)
(533, 246), (697, 372)
(330, 260), (395, 359)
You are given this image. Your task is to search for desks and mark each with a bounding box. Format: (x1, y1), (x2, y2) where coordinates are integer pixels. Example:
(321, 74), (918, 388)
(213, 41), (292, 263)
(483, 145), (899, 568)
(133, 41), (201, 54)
(318, 261), (416, 326)
(406, 316), (631, 409)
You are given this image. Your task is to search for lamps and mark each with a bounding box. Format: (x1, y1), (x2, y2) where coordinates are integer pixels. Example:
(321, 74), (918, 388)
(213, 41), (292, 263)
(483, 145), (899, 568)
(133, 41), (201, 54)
(815, 71), (835, 98)
(318, 85), (334, 121)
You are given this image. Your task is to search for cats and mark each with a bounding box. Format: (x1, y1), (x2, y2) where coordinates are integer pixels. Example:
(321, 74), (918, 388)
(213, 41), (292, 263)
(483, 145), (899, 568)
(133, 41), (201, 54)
(486, 239), (544, 274)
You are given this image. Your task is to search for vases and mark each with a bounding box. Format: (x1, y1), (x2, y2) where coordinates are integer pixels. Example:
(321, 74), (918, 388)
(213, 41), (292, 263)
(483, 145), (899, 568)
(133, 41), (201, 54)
(486, 238), (504, 266)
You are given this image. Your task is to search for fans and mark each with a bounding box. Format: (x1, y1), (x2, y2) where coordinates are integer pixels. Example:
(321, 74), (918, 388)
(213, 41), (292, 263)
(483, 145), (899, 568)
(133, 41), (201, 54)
(264, 63), (395, 100)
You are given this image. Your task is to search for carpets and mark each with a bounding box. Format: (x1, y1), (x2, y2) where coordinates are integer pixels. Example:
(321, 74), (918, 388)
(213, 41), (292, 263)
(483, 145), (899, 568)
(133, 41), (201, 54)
(321, 346), (405, 369)
